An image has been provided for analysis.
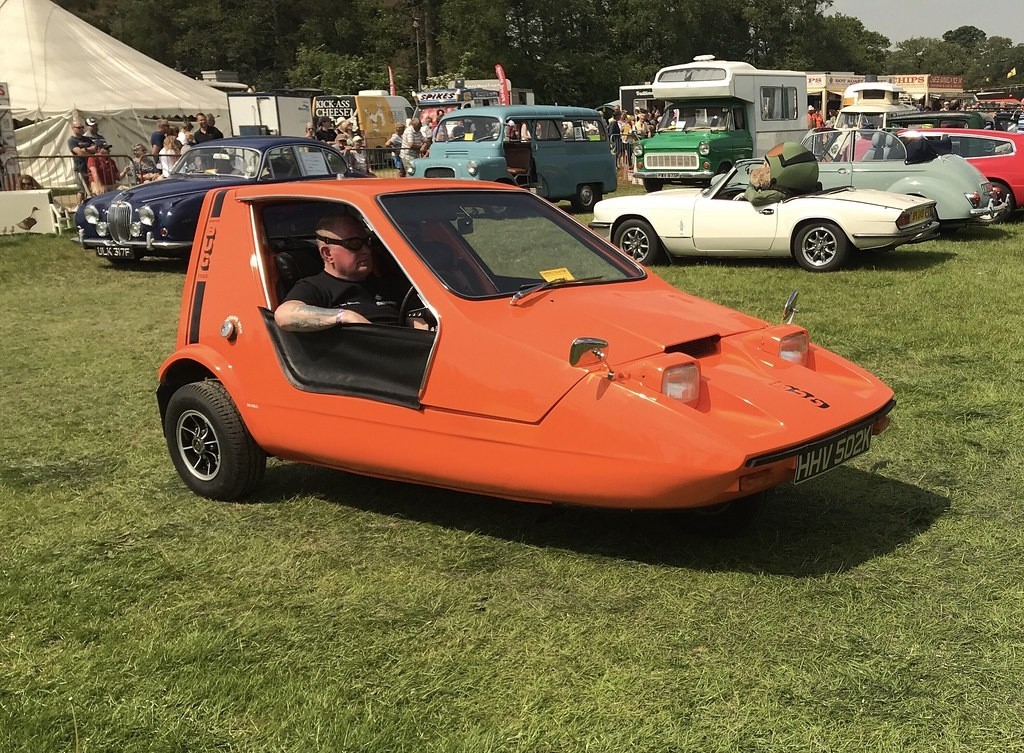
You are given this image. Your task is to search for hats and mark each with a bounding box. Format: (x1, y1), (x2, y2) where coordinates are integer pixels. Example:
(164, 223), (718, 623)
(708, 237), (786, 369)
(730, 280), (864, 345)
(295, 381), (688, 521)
(808, 105), (815, 110)
(85, 118), (99, 126)
(335, 117), (347, 125)
(157, 119), (172, 126)
(95, 139), (112, 147)
(613, 112), (619, 116)
(944, 102), (949, 104)
(318, 116), (329, 127)
(338, 134), (347, 141)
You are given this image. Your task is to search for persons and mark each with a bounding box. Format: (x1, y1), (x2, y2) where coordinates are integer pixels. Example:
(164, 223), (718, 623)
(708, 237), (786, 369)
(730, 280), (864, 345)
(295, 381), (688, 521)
(69, 96), (1024, 204)
(273, 213), (430, 332)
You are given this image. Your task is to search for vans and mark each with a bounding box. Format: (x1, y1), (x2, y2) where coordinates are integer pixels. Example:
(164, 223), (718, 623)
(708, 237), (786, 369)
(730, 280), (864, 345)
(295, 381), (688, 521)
(404, 104), (618, 214)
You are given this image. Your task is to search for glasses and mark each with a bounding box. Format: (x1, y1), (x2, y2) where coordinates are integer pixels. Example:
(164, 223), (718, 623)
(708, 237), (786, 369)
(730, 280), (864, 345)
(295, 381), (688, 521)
(326, 235), (375, 250)
(73, 126), (84, 129)
(308, 128), (314, 131)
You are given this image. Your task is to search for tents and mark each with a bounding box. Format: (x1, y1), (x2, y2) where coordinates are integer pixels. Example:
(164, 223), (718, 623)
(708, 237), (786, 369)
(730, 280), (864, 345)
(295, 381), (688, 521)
(0, 0), (227, 212)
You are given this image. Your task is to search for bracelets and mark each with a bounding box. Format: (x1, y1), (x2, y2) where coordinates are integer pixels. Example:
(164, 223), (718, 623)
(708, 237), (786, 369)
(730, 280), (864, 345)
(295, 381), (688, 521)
(335, 308), (345, 327)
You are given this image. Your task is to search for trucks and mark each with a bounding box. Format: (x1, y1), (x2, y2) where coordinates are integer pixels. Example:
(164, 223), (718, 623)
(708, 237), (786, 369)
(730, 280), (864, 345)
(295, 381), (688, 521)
(630, 53), (816, 193)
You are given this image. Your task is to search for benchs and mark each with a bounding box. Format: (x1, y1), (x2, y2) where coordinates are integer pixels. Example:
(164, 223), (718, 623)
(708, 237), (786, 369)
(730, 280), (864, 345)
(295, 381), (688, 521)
(264, 224), (488, 333)
(501, 148), (532, 184)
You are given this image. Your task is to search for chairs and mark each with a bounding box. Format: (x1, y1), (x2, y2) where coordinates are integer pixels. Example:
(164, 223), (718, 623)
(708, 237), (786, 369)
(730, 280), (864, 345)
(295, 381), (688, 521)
(861, 132), (899, 161)
(78, 172), (93, 202)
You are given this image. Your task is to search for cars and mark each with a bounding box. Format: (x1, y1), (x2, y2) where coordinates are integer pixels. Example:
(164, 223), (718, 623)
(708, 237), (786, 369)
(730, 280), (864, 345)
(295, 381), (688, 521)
(156, 176), (900, 518)
(586, 156), (944, 274)
(69, 133), (379, 265)
(707, 79), (1024, 235)
